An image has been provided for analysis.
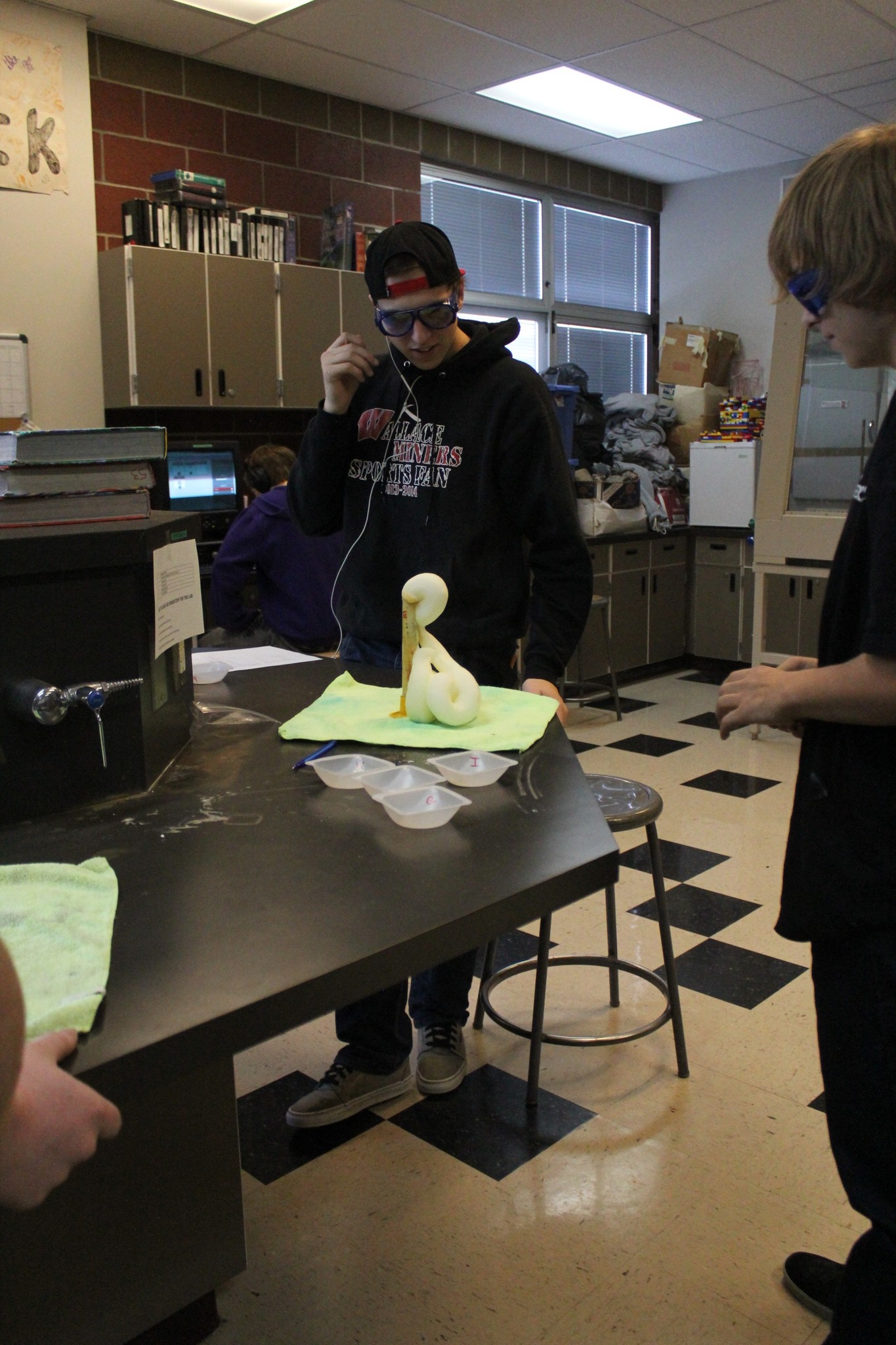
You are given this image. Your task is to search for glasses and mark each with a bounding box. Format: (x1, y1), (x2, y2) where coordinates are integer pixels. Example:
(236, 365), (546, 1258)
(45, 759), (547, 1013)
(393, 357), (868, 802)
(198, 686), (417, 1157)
(374, 288), (459, 337)
(786, 267), (832, 317)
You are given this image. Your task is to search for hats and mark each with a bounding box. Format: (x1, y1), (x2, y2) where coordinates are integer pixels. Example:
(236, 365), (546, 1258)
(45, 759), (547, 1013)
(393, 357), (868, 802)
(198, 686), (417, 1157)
(365, 219), (466, 301)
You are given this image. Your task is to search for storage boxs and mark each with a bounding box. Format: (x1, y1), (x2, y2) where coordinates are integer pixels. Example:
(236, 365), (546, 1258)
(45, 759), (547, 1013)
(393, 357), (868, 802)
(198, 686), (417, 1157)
(655, 320), (762, 529)
(548, 382), (579, 462)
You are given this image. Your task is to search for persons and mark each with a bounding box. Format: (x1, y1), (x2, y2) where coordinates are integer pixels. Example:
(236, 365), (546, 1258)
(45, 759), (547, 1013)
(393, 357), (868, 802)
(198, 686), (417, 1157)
(713, 118), (896, 1344)
(0, 940), (127, 1216)
(195, 443), (353, 655)
(285, 217), (597, 1132)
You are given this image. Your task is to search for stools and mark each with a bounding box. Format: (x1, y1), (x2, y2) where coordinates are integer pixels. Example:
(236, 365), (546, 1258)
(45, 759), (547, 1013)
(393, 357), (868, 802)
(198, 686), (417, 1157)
(552, 594), (623, 722)
(472, 774), (693, 1120)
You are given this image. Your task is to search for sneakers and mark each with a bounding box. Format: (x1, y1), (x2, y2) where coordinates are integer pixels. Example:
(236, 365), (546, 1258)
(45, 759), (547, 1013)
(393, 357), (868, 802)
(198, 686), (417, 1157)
(415, 1022), (467, 1095)
(783, 1251), (846, 1321)
(285, 1055), (414, 1128)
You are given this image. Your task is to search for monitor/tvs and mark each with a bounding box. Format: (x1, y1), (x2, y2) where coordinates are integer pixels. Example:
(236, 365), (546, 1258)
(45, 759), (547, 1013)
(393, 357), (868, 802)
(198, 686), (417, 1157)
(164, 447), (237, 511)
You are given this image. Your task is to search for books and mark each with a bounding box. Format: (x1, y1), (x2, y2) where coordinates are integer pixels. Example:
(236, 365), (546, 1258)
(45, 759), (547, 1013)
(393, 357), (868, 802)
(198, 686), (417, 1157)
(118, 199), (297, 265)
(351, 229), (367, 273)
(151, 168), (228, 211)
(364, 231), (381, 251)
(319, 203), (354, 270)
(0, 425), (167, 527)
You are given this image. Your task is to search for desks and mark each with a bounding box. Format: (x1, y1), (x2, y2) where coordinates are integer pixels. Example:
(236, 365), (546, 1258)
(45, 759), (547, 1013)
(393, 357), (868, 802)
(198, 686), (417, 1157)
(0, 647), (620, 1344)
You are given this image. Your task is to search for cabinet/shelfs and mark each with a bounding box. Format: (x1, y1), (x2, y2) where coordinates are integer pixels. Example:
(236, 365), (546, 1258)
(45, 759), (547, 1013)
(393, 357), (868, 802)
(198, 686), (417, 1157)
(761, 573), (828, 659)
(566, 543), (611, 682)
(691, 536), (754, 666)
(278, 255), (393, 409)
(611, 535), (687, 676)
(96, 243), (281, 411)
